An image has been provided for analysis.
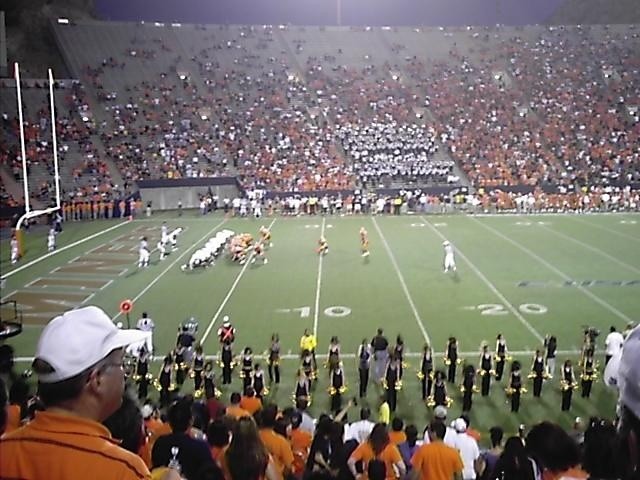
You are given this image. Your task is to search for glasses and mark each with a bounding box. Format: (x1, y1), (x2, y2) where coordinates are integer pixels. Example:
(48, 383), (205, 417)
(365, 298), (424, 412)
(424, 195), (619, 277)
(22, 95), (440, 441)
(85, 356), (135, 388)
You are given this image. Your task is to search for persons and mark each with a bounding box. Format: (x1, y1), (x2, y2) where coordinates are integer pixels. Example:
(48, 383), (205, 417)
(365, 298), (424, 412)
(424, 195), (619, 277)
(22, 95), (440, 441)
(86, 23), (365, 220)
(442, 240), (456, 272)
(319, 235), (329, 255)
(139, 221), (183, 266)
(1, 304), (640, 479)
(10, 234), (19, 263)
(359, 227), (369, 256)
(338, 22), (640, 215)
(47, 226), (56, 253)
(181, 225), (273, 272)
(1, 76), (141, 233)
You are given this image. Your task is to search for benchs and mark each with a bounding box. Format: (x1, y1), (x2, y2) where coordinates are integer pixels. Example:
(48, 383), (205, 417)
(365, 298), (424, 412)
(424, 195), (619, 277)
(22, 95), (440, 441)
(1, 14), (639, 224)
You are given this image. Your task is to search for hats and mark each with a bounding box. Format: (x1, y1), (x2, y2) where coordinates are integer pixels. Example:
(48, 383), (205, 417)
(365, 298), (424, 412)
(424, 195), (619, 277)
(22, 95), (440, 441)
(435, 405), (448, 417)
(451, 418), (466, 430)
(603, 324), (639, 418)
(32, 306), (152, 382)
